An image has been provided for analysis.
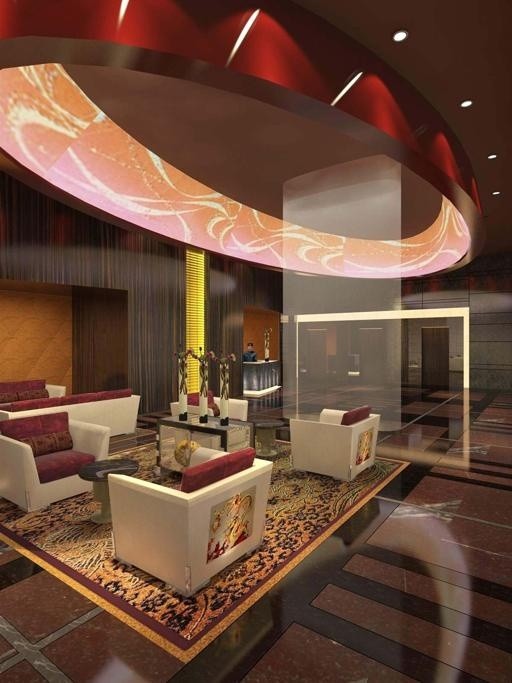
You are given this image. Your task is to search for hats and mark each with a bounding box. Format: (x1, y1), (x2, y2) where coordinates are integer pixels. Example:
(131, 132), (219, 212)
(248, 343), (253, 346)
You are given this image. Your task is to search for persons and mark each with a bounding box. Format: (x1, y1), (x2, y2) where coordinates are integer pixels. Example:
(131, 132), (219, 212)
(244, 343), (256, 362)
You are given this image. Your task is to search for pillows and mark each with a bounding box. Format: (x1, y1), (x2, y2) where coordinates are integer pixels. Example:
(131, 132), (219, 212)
(17, 389), (31, 400)
(30, 433), (54, 457)
(29, 389), (48, 399)
(0, 392), (17, 402)
(31, 434), (57, 456)
(18, 436), (35, 456)
(209, 403), (221, 417)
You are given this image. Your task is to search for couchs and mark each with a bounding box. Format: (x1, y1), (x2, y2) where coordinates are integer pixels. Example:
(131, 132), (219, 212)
(0, 378), (66, 403)
(108, 447), (273, 598)
(290, 404), (380, 481)
(168, 396), (248, 421)
(0, 411), (110, 513)
(0, 387), (141, 437)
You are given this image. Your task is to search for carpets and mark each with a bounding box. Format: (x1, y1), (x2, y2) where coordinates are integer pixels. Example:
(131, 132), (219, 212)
(0, 440), (411, 666)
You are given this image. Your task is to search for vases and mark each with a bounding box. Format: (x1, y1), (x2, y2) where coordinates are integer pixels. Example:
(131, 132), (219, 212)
(198, 359), (207, 422)
(219, 363), (229, 426)
(264, 333), (270, 361)
(177, 357), (187, 421)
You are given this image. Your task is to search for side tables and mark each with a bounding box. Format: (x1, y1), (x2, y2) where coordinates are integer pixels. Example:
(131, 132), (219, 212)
(79, 458), (140, 524)
(248, 417), (284, 456)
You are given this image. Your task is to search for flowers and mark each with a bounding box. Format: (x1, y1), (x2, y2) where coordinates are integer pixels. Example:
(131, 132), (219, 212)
(209, 349), (236, 400)
(264, 327), (273, 349)
(191, 346), (218, 397)
(171, 349), (191, 395)
